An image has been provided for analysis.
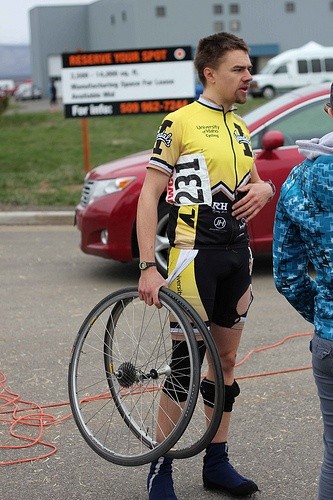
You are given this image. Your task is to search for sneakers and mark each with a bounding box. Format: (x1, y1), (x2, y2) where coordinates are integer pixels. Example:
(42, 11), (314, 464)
(147, 465), (178, 500)
(203, 459), (258, 496)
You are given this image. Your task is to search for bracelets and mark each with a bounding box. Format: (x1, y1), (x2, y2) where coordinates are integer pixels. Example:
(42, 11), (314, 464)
(266, 181), (276, 199)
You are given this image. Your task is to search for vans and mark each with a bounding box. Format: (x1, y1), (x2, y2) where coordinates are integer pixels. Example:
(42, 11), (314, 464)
(249, 47), (333, 102)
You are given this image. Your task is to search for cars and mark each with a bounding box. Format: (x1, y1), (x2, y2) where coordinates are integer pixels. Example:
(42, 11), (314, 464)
(73, 81), (333, 270)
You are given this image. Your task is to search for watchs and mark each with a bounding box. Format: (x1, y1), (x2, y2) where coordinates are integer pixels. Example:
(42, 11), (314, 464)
(139, 261), (157, 270)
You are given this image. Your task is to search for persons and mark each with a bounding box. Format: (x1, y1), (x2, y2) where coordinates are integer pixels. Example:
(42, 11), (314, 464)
(135, 31), (277, 500)
(272, 83), (333, 500)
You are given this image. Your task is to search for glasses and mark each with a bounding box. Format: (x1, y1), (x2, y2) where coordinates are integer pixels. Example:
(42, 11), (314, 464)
(324, 102), (333, 114)
